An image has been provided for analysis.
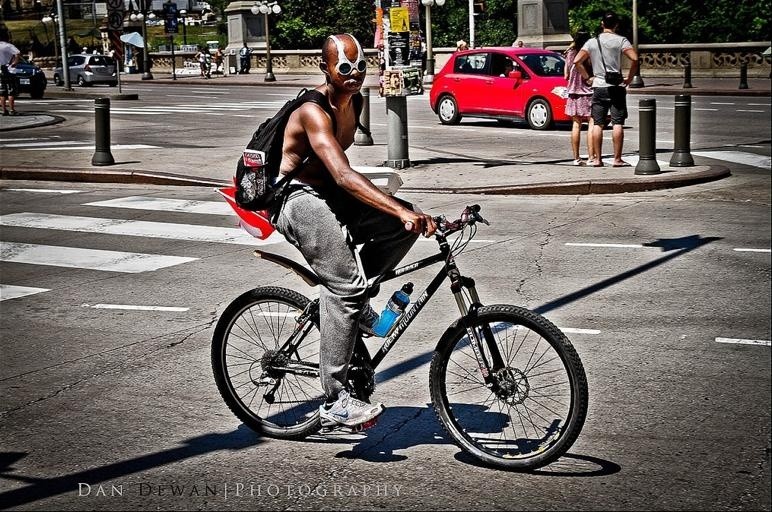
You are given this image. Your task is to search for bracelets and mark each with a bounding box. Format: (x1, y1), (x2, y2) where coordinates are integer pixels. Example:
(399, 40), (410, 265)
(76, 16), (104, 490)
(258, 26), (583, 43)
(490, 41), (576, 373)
(585, 76), (590, 81)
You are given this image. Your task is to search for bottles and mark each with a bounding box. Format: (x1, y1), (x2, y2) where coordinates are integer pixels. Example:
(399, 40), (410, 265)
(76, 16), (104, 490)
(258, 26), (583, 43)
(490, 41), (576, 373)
(370, 281), (415, 338)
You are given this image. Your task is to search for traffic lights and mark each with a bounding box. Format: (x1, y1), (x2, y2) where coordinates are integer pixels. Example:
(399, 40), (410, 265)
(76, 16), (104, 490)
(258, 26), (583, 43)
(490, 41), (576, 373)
(473, 1), (488, 19)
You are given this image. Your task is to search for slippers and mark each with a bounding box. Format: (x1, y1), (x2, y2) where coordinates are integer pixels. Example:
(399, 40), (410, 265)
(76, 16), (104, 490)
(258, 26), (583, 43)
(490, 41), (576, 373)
(613, 162), (632, 167)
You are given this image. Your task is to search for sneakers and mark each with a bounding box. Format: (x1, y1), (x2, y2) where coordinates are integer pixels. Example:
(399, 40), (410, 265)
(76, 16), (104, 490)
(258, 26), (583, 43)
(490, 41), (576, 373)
(359, 304), (383, 338)
(319, 391), (384, 427)
(574, 157), (603, 167)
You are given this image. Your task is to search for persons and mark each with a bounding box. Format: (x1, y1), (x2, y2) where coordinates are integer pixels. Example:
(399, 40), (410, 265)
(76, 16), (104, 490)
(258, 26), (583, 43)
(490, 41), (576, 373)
(194, 45), (224, 79)
(573, 12), (639, 167)
(563, 28), (596, 166)
(235, 33), (437, 433)
(0, 29), (22, 116)
(80, 44), (115, 56)
(457, 40), (545, 77)
(239, 43), (252, 74)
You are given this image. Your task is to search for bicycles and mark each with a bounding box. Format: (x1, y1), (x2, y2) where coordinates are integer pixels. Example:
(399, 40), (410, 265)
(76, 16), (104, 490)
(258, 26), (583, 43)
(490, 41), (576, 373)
(210, 206), (590, 471)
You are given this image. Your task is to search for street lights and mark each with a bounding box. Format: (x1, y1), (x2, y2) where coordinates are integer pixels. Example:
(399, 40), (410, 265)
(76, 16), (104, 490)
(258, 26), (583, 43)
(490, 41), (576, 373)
(130, 8), (158, 81)
(421, 0), (447, 85)
(42, 11), (61, 66)
(250, 0), (282, 82)
(179, 8), (188, 46)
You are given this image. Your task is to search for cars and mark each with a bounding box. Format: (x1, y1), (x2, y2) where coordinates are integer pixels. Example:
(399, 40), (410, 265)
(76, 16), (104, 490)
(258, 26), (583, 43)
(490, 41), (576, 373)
(428, 45), (613, 131)
(52, 53), (119, 88)
(4, 53), (48, 100)
(145, 15), (198, 25)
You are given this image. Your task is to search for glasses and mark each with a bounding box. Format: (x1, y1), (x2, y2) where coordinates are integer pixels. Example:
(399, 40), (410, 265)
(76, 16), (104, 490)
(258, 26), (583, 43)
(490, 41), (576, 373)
(326, 33), (367, 76)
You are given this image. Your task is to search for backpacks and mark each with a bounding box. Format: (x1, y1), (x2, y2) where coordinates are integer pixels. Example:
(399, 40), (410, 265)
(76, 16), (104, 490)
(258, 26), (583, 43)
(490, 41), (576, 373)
(235, 91), (363, 211)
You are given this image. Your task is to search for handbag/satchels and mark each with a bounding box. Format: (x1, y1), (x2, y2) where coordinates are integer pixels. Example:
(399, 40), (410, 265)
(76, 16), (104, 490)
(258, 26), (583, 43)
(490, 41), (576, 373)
(605, 71), (624, 85)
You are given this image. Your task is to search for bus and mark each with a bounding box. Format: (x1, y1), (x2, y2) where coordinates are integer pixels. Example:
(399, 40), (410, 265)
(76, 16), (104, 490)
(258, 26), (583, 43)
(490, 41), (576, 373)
(206, 40), (220, 51)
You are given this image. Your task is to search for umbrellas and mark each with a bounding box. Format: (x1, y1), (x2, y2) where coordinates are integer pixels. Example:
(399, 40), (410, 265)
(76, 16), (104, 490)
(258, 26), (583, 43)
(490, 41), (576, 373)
(119, 32), (152, 48)
